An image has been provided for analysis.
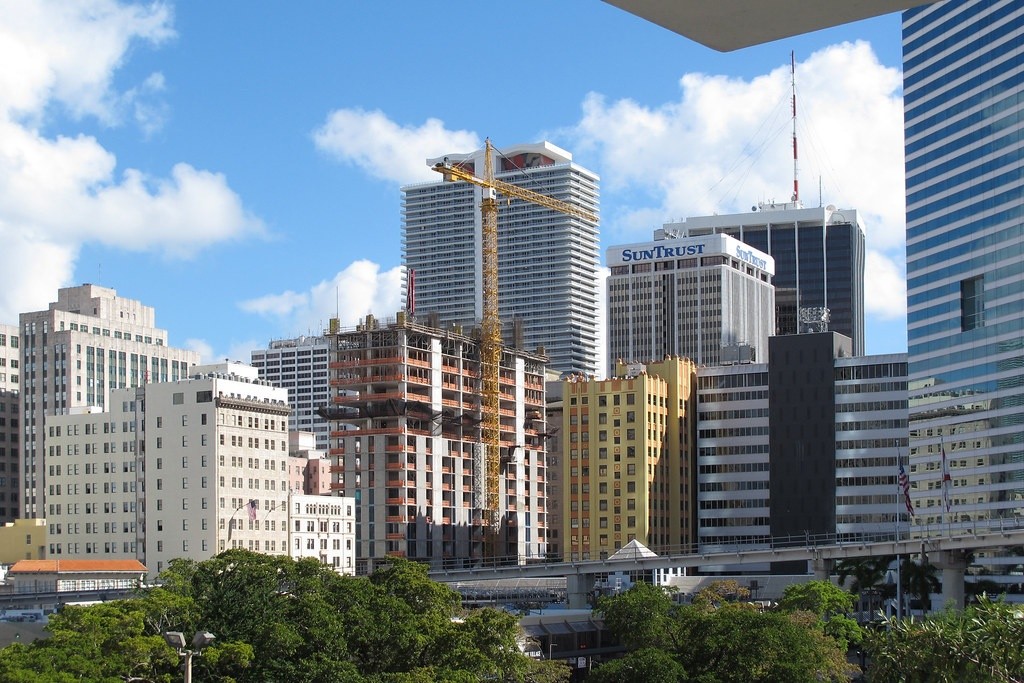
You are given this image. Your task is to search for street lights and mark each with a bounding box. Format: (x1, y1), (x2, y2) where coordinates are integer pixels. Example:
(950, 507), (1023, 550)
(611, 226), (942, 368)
(165, 631), (216, 683)
(549, 644), (559, 660)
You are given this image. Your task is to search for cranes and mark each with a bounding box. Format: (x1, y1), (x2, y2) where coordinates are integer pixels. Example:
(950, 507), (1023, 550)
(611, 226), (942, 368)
(432, 138), (602, 564)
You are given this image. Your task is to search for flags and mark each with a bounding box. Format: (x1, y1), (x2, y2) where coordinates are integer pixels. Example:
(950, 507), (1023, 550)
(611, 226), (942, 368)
(942, 448), (955, 511)
(899, 455), (914, 517)
(247, 501), (256, 521)
